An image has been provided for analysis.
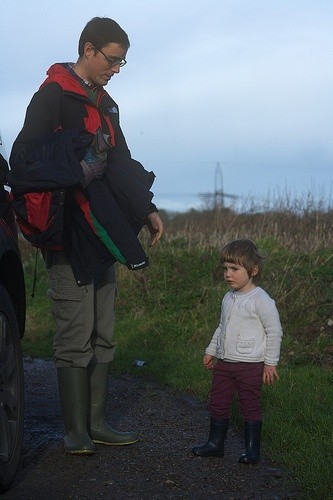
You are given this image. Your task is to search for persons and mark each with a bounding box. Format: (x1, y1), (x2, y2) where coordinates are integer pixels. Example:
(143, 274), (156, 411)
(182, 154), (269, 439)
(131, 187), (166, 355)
(192, 239), (283, 464)
(8, 16), (164, 453)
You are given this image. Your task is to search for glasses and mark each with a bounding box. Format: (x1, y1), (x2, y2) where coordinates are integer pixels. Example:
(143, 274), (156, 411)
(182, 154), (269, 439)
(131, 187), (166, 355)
(92, 44), (127, 68)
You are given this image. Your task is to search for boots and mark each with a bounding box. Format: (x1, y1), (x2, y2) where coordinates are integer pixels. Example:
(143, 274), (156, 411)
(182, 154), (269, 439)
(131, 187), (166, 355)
(56, 365), (97, 456)
(87, 363), (140, 446)
(237, 420), (263, 465)
(192, 416), (230, 458)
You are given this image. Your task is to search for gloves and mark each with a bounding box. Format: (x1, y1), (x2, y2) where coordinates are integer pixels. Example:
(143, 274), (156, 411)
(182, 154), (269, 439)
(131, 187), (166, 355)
(81, 149), (107, 182)
